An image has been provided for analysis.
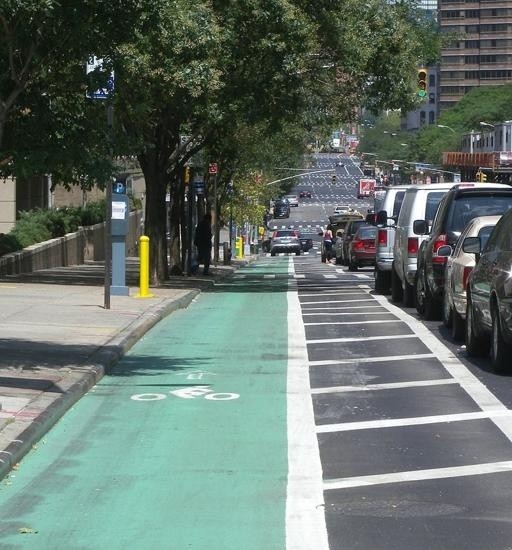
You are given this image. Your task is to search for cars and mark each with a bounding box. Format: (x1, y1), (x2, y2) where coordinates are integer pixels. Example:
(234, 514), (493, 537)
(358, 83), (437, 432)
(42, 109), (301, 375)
(268, 229), (302, 255)
(272, 190), (311, 218)
(411, 184), (512, 372)
(321, 205), (378, 271)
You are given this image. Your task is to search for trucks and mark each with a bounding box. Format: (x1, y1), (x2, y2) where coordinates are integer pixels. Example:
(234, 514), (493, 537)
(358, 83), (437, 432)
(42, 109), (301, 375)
(356, 179), (377, 199)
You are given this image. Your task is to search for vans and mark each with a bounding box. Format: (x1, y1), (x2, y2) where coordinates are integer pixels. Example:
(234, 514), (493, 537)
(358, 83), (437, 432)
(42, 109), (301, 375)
(373, 182), (459, 310)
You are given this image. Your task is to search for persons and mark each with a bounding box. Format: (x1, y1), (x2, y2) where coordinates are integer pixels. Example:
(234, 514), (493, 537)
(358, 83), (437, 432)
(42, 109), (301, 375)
(322, 224), (335, 264)
(191, 214), (214, 276)
